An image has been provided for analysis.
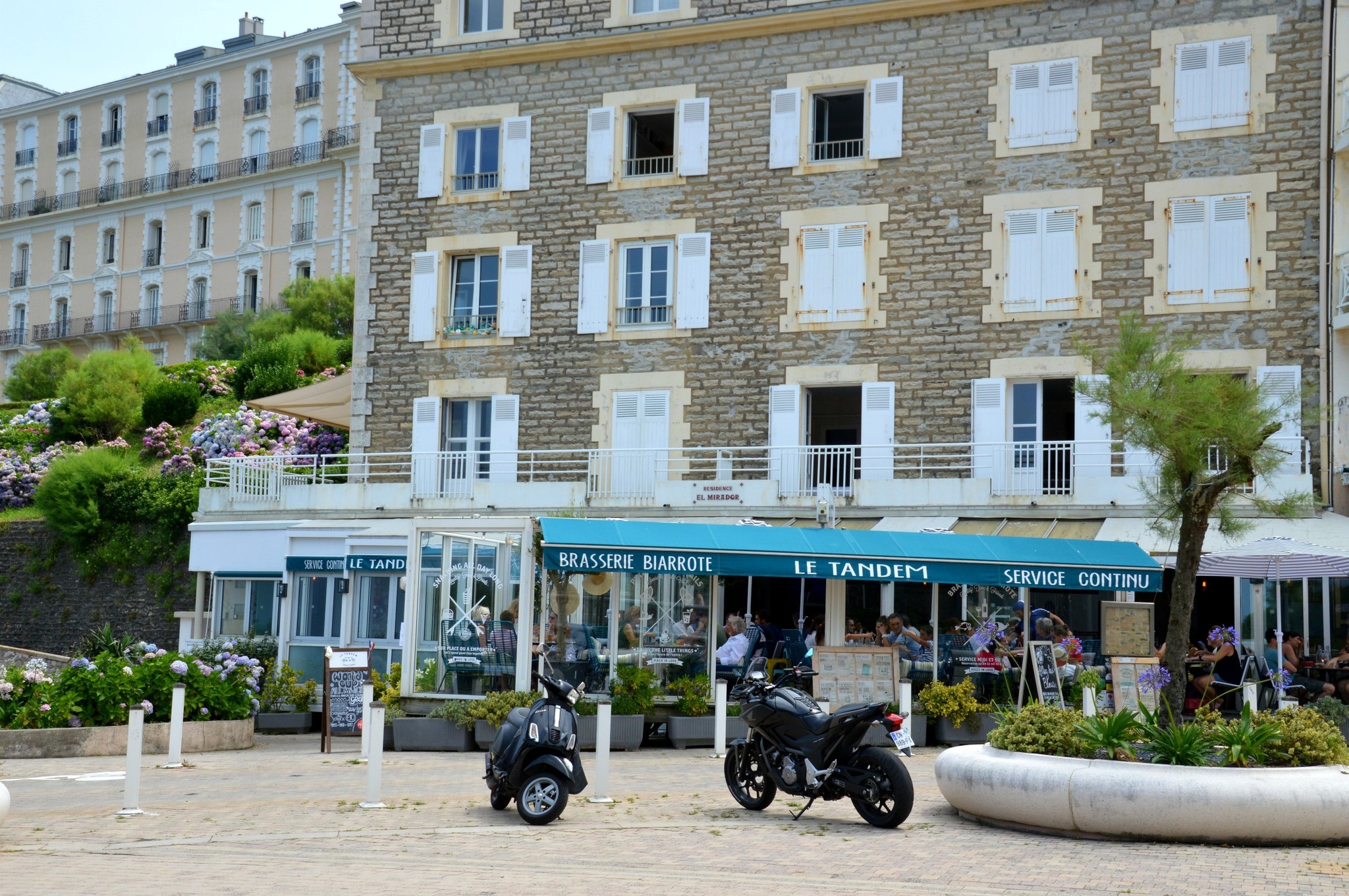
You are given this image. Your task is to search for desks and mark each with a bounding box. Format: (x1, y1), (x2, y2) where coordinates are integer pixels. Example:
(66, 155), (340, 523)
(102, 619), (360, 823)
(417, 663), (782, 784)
(1183, 661), (1209, 697)
(531, 642), (577, 644)
(644, 644), (703, 695)
(1304, 666), (1349, 683)
(1009, 656), (1033, 703)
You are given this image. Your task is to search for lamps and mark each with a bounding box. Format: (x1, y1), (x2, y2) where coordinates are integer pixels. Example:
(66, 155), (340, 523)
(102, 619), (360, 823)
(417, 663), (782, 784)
(400, 576), (406, 593)
(276, 583), (288, 597)
(337, 578), (349, 593)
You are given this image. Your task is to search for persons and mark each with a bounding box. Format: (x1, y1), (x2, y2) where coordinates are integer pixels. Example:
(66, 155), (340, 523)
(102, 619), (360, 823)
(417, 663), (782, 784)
(1154, 642), (1166, 661)
(942, 600), (1082, 704)
(508, 598), (538, 642)
(466, 606), (491, 663)
(1262, 624), (1349, 704)
(802, 614), (825, 651)
(618, 607), (655, 671)
(489, 610), (538, 659)
(667, 605), (727, 684)
(1187, 626), (1242, 713)
(546, 610), (577, 661)
(845, 613), (933, 672)
(687, 611), (784, 681)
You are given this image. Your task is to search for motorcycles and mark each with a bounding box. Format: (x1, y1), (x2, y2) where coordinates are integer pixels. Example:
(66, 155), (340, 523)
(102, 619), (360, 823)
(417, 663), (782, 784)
(482, 643), (588, 826)
(725, 648), (914, 828)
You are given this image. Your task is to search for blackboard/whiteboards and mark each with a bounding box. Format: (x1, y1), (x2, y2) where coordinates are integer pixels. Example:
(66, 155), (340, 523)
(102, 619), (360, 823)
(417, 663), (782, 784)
(1018, 641), (1065, 711)
(321, 647), (372, 736)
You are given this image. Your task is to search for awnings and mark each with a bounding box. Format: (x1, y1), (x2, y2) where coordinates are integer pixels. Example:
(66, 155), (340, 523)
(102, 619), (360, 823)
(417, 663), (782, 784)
(245, 371), (351, 431)
(534, 516), (1163, 592)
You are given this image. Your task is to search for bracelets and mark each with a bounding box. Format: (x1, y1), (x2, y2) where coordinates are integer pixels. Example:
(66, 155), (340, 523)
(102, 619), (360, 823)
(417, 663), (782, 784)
(1199, 654), (1203, 661)
(643, 634), (647, 639)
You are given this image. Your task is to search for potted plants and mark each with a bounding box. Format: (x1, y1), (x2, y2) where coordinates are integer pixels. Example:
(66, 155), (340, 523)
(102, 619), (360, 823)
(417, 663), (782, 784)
(378, 700), (406, 751)
(661, 674), (754, 749)
(253, 657), (319, 735)
(1302, 695), (1349, 745)
(568, 664), (664, 750)
(467, 689), (543, 749)
(860, 700), (928, 748)
(391, 699), (474, 751)
(918, 675), (1012, 742)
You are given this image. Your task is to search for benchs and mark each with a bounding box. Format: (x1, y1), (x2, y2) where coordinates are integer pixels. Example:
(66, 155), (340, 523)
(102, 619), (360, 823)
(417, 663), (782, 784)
(437, 619), (1307, 715)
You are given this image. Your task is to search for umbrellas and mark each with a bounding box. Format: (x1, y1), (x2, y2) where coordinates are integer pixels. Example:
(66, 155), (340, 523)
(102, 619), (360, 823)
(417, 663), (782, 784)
(1162, 536), (1349, 711)
